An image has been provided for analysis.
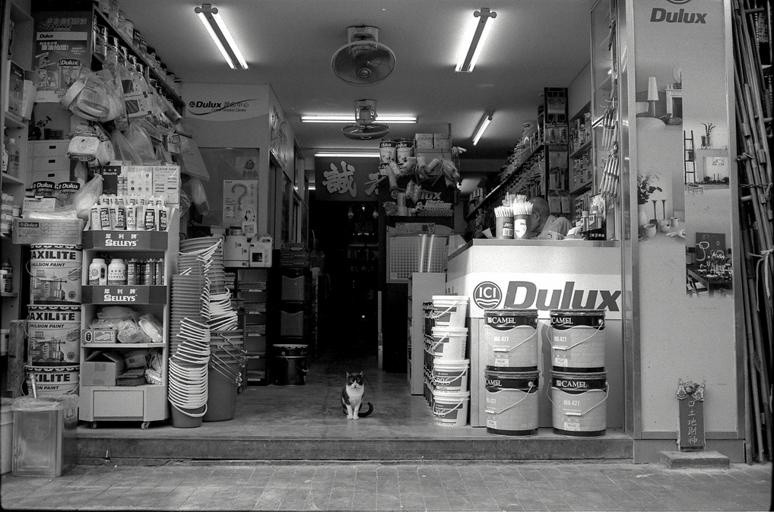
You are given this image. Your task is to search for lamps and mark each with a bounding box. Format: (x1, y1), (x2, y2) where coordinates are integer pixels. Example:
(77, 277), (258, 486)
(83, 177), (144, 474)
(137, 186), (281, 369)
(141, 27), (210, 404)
(194, 2), (249, 71)
(456, 7), (497, 73)
(470, 114), (496, 146)
(298, 112), (418, 126)
(646, 76), (660, 117)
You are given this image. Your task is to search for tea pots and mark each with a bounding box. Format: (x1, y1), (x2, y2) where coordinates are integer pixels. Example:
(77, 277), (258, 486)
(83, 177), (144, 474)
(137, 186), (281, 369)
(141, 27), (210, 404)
(660, 220), (670, 232)
(670, 216), (680, 228)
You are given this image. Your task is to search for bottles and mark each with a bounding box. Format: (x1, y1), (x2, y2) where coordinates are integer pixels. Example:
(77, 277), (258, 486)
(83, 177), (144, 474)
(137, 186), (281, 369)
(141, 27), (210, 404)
(86, 256), (163, 287)
(579, 206), (602, 232)
(8, 20), (15, 60)
(1, 134), (18, 180)
(548, 127), (565, 145)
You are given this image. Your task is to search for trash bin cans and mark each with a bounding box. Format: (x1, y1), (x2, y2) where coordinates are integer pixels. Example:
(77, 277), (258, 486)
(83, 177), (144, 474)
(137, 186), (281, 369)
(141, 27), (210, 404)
(11, 392), (79, 478)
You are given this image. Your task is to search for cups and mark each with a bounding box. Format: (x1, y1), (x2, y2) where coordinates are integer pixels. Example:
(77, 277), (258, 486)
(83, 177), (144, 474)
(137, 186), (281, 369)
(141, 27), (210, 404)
(643, 223), (656, 238)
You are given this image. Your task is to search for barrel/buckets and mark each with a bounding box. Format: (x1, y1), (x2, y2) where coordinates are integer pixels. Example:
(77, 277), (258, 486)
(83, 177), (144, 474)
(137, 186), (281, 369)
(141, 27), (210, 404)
(546, 369), (612, 438)
(430, 389), (471, 427)
(482, 308), (539, 371)
(430, 328), (469, 363)
(26, 303), (81, 366)
(166, 235), (251, 430)
(543, 307), (608, 372)
(431, 359), (470, 393)
(271, 342), (309, 387)
(0, 398), (15, 476)
(23, 364), (79, 431)
(24, 242), (82, 304)
(427, 294), (469, 327)
(249, 235), (273, 269)
(481, 370), (541, 437)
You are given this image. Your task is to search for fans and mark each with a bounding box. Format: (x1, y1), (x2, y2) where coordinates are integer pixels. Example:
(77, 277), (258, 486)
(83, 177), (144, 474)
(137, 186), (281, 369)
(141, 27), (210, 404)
(342, 99), (392, 141)
(330, 24), (395, 86)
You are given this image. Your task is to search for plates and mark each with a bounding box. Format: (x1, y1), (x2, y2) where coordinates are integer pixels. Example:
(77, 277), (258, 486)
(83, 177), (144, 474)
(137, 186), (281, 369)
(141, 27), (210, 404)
(696, 268), (707, 273)
(705, 274), (718, 278)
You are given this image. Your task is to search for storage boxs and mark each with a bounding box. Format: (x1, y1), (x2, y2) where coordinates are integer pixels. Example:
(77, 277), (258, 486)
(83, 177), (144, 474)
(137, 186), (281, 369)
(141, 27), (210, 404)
(82, 350), (123, 387)
(415, 132), (452, 177)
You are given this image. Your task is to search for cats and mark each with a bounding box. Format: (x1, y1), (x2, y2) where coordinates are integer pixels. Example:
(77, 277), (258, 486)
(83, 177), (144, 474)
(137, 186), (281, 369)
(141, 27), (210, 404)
(341, 371), (373, 420)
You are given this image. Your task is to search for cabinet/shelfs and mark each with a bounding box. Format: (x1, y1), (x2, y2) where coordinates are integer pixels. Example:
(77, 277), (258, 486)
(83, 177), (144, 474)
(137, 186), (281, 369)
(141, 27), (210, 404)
(465, 134), (594, 237)
(224, 265), (277, 385)
(77, 208), (179, 427)
(0, 2), (32, 404)
(90, 6), (185, 129)
(404, 273), (447, 396)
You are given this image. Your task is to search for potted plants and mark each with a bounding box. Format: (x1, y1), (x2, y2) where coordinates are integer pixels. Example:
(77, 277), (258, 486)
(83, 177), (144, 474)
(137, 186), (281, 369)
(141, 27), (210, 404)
(700, 122), (716, 148)
(637, 171), (664, 235)
